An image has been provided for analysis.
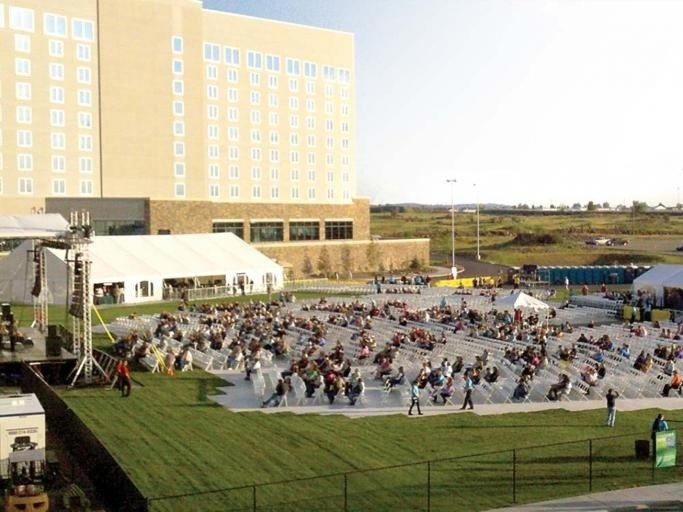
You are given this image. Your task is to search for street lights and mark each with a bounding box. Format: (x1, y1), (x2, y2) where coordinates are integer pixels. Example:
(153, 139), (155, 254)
(445, 178), (459, 280)
(471, 182), (481, 261)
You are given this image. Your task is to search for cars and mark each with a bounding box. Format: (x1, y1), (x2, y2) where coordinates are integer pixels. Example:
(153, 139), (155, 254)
(583, 234), (612, 246)
(610, 237), (628, 247)
(674, 245), (682, 251)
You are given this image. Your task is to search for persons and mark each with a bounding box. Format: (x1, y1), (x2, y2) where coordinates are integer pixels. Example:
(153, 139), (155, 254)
(649, 411), (668, 457)
(92, 268), (683, 406)
(407, 378), (423, 416)
(605, 387), (619, 427)
(11, 318), (24, 336)
(459, 371), (474, 410)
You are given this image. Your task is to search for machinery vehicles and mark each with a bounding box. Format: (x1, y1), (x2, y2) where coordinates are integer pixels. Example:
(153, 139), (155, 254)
(1, 440), (51, 512)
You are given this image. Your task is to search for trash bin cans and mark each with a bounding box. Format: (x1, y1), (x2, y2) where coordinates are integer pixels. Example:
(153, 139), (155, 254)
(635, 440), (650, 461)
